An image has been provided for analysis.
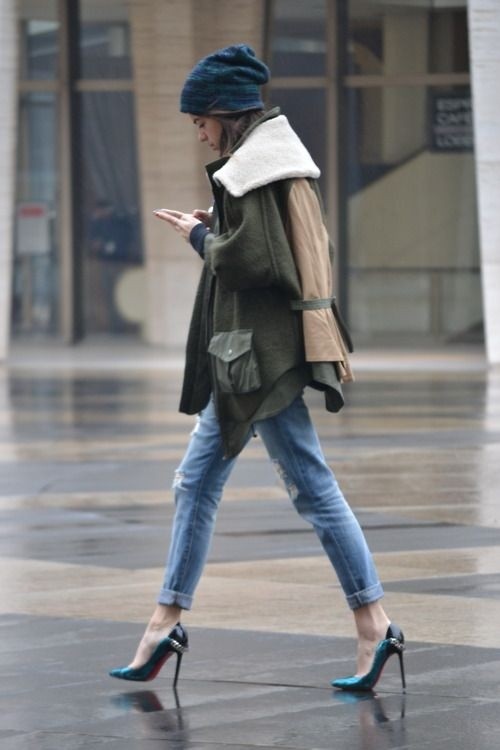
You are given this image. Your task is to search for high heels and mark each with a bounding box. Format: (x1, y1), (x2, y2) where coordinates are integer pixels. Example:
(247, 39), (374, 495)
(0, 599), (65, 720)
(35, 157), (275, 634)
(329, 621), (407, 691)
(108, 621), (189, 688)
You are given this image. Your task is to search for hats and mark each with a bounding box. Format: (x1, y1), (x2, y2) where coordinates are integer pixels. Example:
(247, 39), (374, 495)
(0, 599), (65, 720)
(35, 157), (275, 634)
(179, 42), (272, 114)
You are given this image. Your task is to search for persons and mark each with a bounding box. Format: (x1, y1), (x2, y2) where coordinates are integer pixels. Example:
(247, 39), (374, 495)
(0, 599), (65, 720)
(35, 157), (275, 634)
(109, 40), (407, 692)
